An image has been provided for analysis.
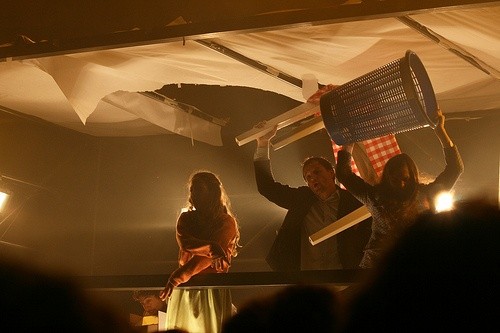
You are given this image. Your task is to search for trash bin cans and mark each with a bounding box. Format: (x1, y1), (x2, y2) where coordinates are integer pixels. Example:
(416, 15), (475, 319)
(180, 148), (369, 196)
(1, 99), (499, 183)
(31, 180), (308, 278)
(319, 50), (438, 146)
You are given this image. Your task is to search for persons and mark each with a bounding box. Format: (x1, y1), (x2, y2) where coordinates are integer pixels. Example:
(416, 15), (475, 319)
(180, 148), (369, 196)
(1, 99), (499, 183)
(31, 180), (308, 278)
(133, 288), (167, 317)
(156, 169), (239, 333)
(333, 105), (464, 295)
(253, 118), (373, 294)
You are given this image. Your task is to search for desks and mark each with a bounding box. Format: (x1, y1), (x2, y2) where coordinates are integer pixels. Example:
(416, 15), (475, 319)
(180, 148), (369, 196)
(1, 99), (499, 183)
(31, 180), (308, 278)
(232, 91), (381, 247)
